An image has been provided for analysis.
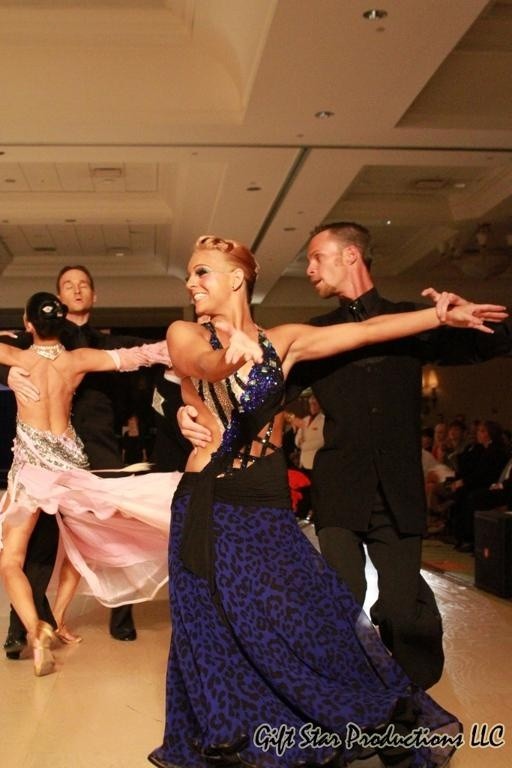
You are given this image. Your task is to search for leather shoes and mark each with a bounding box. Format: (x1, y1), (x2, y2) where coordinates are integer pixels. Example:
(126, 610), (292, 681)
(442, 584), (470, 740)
(110, 607), (135, 641)
(5, 640), (23, 660)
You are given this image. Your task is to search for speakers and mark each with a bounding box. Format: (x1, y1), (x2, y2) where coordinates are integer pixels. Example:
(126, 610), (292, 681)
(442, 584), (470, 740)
(473, 510), (512, 598)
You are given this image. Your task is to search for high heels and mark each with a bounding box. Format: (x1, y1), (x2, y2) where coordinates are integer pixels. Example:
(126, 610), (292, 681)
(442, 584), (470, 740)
(31, 622), (56, 675)
(57, 626), (83, 644)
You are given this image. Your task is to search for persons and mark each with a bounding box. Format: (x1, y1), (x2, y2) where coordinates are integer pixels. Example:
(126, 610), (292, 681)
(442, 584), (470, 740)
(148, 231), (511, 766)
(0, 264), (157, 660)
(169, 218), (475, 690)
(281, 386), (512, 551)
(121, 415), (146, 463)
(0, 290), (176, 675)
